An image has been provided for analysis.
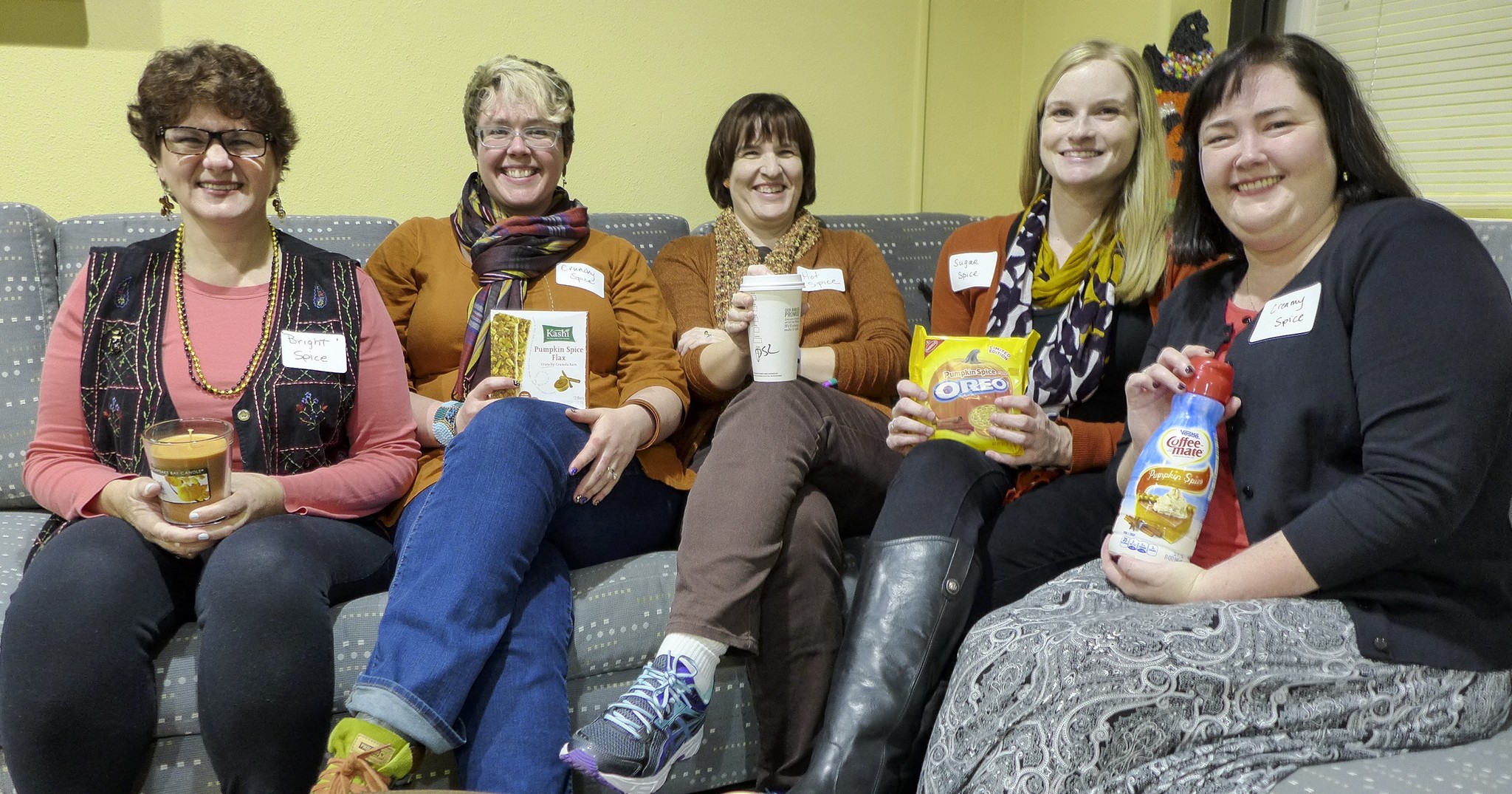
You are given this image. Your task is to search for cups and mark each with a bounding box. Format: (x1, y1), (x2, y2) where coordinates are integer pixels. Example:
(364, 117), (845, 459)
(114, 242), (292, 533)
(142, 416), (236, 529)
(739, 271), (807, 383)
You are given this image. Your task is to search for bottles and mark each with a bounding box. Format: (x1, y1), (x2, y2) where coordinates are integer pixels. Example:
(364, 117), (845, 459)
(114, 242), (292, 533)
(1105, 354), (1238, 567)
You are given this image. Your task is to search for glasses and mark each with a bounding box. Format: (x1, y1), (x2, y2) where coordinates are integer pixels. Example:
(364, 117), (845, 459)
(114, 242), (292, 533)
(477, 123), (562, 154)
(153, 123), (272, 163)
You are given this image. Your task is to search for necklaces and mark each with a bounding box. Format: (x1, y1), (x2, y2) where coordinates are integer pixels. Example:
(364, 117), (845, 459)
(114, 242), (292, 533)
(1243, 223), (1329, 313)
(172, 219), (282, 395)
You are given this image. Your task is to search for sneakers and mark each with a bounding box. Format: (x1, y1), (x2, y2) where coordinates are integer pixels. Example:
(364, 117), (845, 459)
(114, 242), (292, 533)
(295, 719), (427, 791)
(561, 653), (715, 792)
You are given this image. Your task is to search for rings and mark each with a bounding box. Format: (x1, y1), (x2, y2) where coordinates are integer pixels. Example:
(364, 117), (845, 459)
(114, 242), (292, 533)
(891, 418), (903, 433)
(1141, 367), (1148, 375)
(607, 466), (618, 480)
(704, 329), (713, 338)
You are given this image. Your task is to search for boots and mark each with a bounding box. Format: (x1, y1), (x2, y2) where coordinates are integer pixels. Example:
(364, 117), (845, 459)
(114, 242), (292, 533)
(793, 539), (984, 792)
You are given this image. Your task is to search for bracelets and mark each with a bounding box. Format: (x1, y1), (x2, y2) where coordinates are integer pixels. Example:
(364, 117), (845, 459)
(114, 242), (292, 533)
(620, 398), (661, 452)
(432, 399), (463, 448)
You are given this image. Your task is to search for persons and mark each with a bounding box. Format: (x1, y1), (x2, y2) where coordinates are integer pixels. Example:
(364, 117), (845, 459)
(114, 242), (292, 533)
(787, 39), (1233, 794)
(1, 35), (419, 794)
(919, 30), (1512, 794)
(307, 50), (694, 794)
(558, 90), (916, 794)
